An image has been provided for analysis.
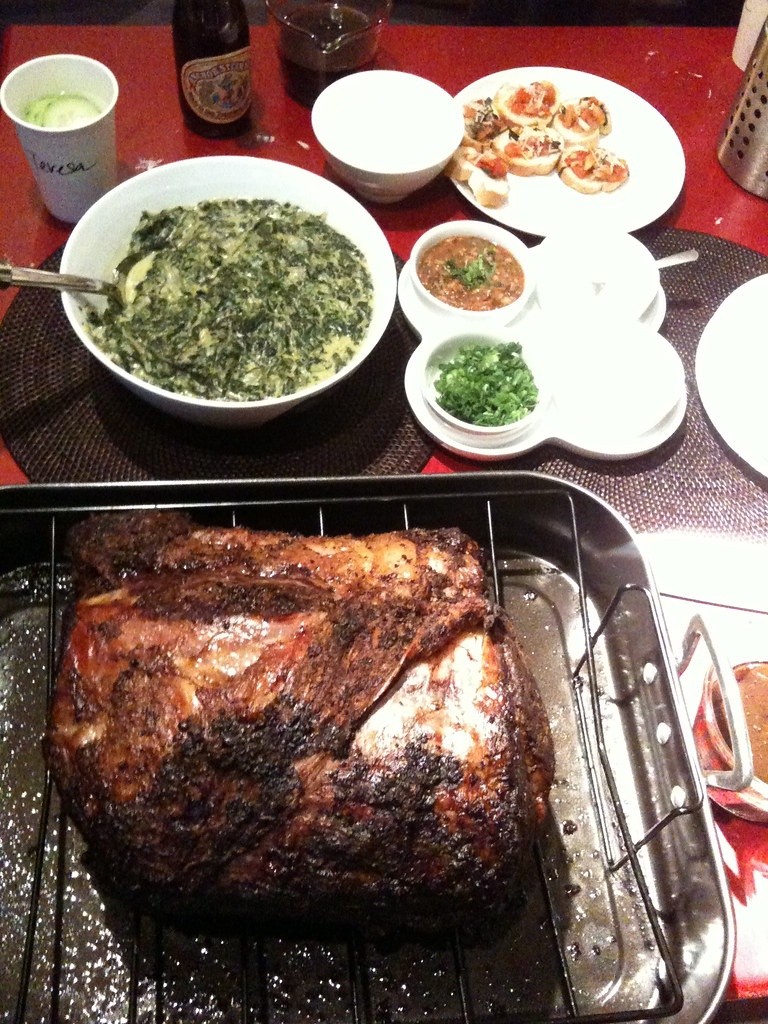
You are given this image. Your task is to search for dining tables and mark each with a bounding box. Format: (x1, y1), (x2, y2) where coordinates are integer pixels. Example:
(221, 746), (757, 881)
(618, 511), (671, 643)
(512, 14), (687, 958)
(3, 21), (766, 1024)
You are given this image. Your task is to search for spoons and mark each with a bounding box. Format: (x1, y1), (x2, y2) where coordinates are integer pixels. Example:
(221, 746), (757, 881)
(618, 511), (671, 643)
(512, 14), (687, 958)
(0, 250), (163, 310)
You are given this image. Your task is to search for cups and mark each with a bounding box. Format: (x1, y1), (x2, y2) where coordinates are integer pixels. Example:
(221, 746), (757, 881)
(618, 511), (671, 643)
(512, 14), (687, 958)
(1, 54), (120, 225)
(267, 0), (390, 108)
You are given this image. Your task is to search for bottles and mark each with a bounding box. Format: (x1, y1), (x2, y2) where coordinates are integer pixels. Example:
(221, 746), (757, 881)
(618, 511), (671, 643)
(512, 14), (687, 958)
(171, 0), (251, 135)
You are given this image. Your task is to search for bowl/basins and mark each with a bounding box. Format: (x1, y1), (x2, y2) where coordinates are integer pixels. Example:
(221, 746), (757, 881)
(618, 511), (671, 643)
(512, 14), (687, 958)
(693, 646), (768, 824)
(58, 155), (398, 429)
(311, 69), (465, 203)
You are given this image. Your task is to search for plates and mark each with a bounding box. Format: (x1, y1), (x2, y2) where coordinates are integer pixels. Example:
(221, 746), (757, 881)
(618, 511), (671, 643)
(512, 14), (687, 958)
(446, 65), (686, 239)
(395, 219), (687, 462)
(695, 273), (768, 481)
